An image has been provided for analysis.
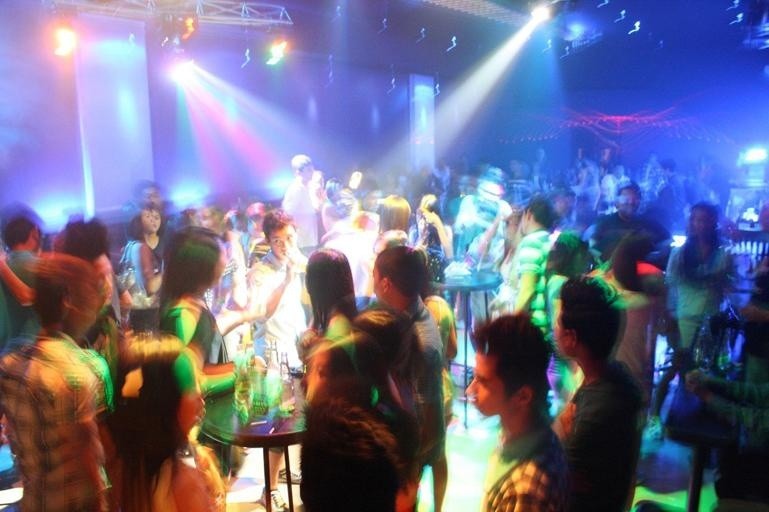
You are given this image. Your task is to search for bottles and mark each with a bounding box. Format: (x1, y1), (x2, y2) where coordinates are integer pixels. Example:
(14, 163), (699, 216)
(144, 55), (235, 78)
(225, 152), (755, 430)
(230, 344), (294, 418)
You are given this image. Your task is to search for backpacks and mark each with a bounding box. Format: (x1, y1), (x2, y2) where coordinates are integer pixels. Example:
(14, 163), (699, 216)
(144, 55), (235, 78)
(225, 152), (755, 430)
(114, 260), (160, 312)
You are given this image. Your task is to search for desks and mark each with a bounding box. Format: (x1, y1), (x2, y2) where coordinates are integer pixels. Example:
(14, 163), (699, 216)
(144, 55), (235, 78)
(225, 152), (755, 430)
(197, 374), (306, 512)
(432, 271), (502, 427)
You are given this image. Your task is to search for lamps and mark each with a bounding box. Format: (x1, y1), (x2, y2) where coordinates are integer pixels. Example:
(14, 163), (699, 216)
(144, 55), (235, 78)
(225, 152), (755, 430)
(531, 0), (565, 21)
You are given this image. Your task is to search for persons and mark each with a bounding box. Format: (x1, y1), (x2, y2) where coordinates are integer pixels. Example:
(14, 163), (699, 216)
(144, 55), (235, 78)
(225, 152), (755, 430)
(1, 147), (769, 512)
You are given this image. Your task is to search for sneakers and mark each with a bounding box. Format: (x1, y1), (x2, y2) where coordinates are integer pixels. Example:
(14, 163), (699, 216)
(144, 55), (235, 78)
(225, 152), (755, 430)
(278, 468), (301, 483)
(259, 489), (290, 512)
